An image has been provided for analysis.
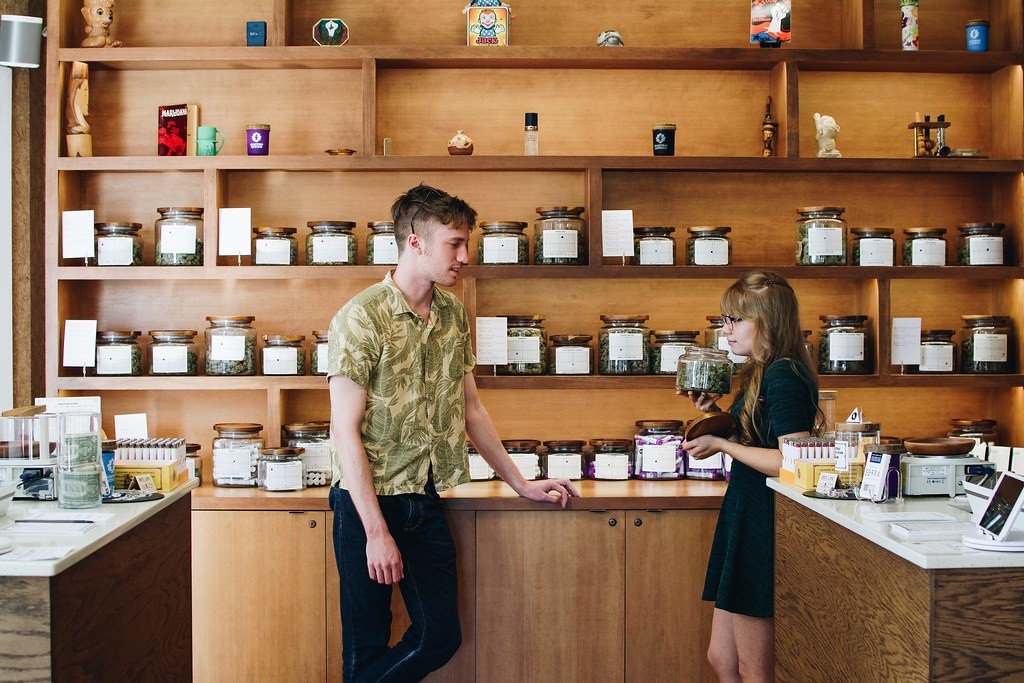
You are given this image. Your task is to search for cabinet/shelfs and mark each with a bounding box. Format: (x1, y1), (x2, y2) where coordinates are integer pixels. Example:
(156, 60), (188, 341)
(191, 508), (475, 683)
(45, 0), (1024, 450)
(476, 507), (722, 683)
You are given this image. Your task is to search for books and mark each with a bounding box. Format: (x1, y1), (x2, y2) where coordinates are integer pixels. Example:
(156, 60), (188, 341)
(750, 0), (791, 43)
(158, 102), (199, 155)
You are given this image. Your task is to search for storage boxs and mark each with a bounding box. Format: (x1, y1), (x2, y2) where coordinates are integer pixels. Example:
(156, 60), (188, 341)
(113, 460), (177, 493)
(796, 459), (865, 490)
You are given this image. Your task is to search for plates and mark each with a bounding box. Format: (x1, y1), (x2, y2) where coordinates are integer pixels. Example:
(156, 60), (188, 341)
(0, 441), (56, 457)
(904, 438), (976, 454)
(325, 149), (356, 155)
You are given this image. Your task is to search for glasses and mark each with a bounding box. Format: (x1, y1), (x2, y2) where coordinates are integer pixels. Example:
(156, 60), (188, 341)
(721, 313), (753, 330)
(411, 186), (442, 234)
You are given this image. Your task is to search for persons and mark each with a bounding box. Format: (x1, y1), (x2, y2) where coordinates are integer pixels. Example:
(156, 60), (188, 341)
(678, 269), (827, 683)
(328, 180), (579, 683)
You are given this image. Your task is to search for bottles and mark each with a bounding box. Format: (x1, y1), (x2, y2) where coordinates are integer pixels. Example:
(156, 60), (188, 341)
(448, 130), (474, 155)
(258, 447), (307, 491)
(306, 221), (358, 266)
(366, 221), (399, 265)
(902, 227), (948, 266)
(850, 227), (897, 266)
(186, 443), (202, 487)
(685, 226), (732, 266)
(154, 207), (204, 266)
(309, 330), (330, 375)
(795, 206), (847, 266)
(956, 222), (1006, 266)
(630, 226), (676, 265)
(204, 315), (256, 375)
(534, 206), (586, 265)
(284, 421), (332, 486)
(211, 422), (265, 487)
(524, 113), (538, 156)
(93, 330), (142, 376)
(91, 221), (144, 266)
(259, 334), (306, 375)
(251, 226), (298, 266)
(477, 221), (530, 265)
(466, 314), (1012, 486)
(146, 330), (197, 375)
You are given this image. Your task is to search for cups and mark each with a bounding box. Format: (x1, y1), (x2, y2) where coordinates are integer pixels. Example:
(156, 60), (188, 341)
(966, 18), (991, 51)
(246, 124), (271, 155)
(652, 122), (676, 156)
(198, 126), (224, 155)
